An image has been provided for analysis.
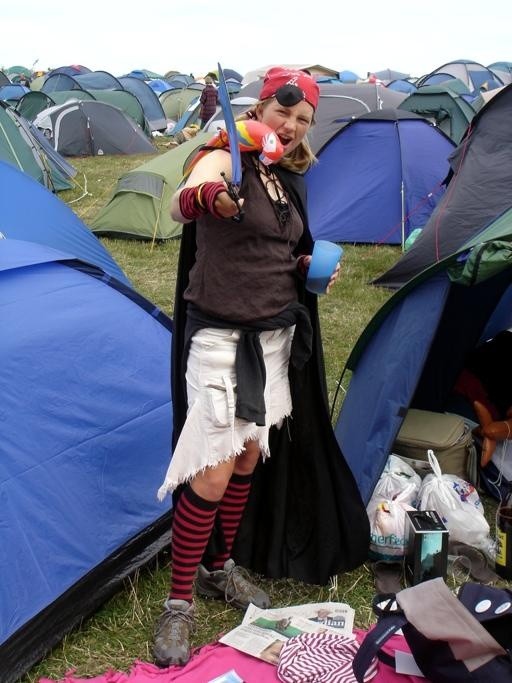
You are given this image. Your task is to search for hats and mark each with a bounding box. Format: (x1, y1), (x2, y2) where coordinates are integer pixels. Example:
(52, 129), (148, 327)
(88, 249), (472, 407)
(259, 66), (320, 113)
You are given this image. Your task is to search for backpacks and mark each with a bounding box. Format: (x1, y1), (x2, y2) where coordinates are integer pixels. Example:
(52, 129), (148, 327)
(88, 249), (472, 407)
(352, 576), (511, 683)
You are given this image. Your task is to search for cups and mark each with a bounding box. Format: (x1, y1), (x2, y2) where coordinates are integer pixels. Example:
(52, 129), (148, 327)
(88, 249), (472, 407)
(447, 555), (472, 579)
(451, 567), (468, 597)
(495, 488), (512, 578)
(305, 238), (341, 298)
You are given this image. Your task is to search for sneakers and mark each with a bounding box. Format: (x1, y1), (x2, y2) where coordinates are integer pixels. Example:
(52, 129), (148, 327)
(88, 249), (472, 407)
(194, 558), (272, 611)
(153, 592), (198, 666)
